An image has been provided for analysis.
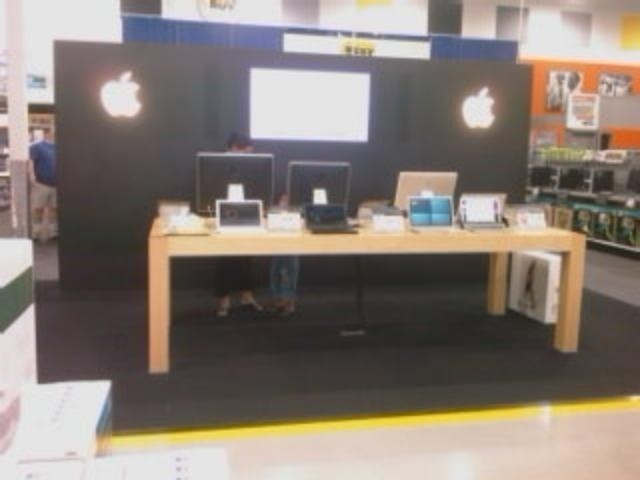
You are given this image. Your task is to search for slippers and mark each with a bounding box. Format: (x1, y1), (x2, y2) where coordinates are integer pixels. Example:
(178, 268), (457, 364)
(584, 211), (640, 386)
(215, 300), (233, 318)
(240, 296), (263, 313)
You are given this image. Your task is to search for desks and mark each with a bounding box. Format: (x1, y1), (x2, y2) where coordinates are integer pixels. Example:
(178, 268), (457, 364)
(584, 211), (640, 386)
(147, 216), (588, 375)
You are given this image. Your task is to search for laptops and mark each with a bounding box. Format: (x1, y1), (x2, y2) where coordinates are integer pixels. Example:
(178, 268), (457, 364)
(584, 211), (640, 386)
(215, 200), (267, 235)
(303, 204), (360, 234)
(408, 197), (465, 233)
(457, 199), (505, 230)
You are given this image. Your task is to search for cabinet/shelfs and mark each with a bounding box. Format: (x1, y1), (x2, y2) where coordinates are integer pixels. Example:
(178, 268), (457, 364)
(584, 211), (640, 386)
(527, 145), (640, 257)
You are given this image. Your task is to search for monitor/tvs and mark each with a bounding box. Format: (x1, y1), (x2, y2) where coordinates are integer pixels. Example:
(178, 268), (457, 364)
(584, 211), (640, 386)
(592, 169), (614, 193)
(529, 165), (557, 187)
(626, 170), (640, 194)
(195, 152), (276, 215)
(393, 172), (459, 210)
(558, 166), (590, 191)
(287, 161), (353, 212)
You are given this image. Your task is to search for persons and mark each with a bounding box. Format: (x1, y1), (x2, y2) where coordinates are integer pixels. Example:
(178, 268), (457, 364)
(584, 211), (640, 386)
(519, 261), (537, 308)
(29, 128), (58, 242)
(216, 132), (263, 317)
(264, 193), (300, 315)
